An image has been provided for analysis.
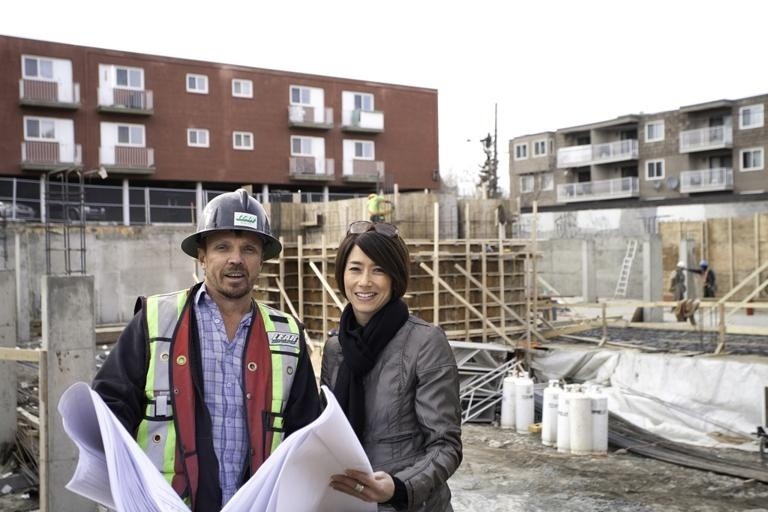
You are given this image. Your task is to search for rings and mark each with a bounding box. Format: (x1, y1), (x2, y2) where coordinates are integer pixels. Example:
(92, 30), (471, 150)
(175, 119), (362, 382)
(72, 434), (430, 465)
(354, 483), (364, 494)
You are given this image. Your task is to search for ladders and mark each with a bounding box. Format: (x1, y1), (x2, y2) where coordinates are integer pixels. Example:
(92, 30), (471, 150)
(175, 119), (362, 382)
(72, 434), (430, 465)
(614, 238), (638, 298)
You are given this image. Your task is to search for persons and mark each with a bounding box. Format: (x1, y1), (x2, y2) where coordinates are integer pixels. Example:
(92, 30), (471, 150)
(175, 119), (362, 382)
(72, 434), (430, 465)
(320, 220), (463, 512)
(685, 259), (717, 298)
(366, 195), (385, 223)
(91, 186), (323, 511)
(667, 261), (687, 301)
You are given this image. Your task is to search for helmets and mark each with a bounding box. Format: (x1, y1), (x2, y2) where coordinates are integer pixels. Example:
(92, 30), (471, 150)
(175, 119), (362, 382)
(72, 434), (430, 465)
(699, 260), (708, 267)
(181, 188), (282, 261)
(677, 261), (686, 268)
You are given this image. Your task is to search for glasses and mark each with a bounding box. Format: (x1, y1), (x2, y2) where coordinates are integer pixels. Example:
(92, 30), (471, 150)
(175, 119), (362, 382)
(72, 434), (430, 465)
(346, 221), (399, 239)
